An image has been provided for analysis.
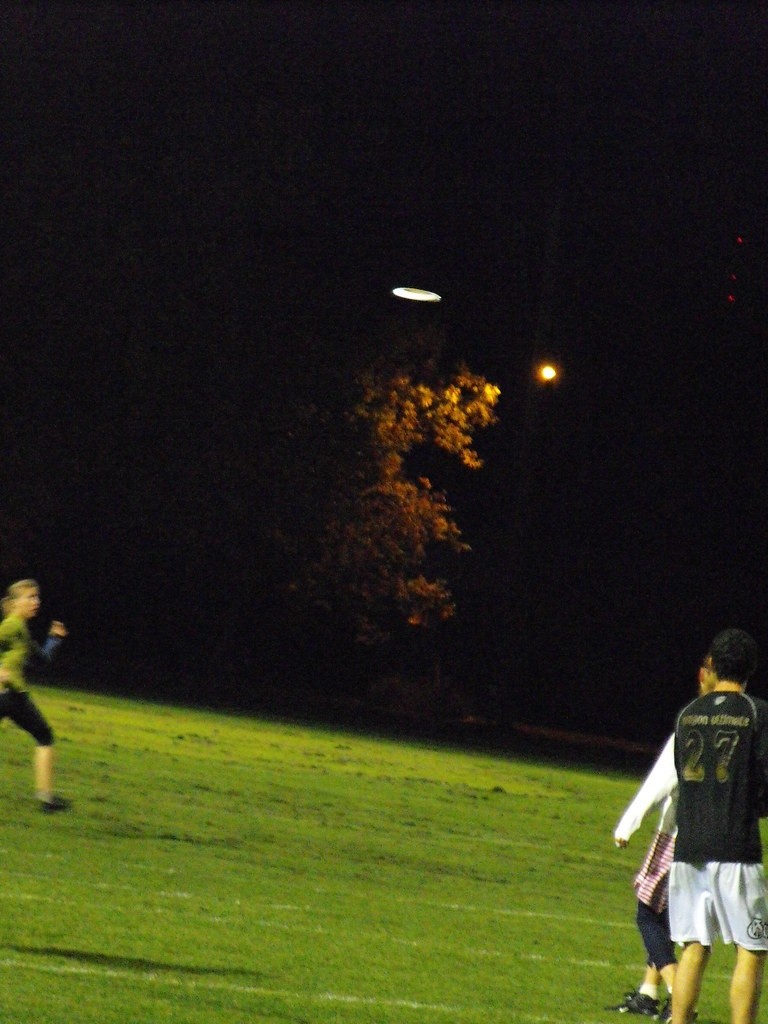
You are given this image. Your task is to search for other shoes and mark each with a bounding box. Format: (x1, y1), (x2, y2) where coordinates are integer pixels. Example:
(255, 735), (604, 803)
(40, 795), (70, 813)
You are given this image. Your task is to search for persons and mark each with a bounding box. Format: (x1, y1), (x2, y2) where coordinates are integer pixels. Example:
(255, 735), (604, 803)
(604, 665), (714, 1024)
(0, 580), (72, 813)
(661, 628), (768, 1024)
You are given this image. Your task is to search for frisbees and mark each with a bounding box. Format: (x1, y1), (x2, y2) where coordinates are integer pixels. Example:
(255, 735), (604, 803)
(391, 287), (441, 303)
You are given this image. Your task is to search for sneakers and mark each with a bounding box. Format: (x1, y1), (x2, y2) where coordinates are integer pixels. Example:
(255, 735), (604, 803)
(607, 986), (661, 1021)
(659, 993), (699, 1024)
